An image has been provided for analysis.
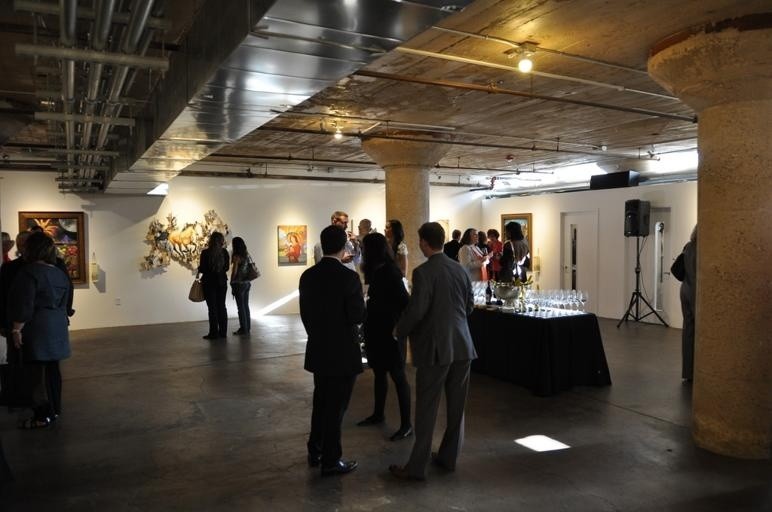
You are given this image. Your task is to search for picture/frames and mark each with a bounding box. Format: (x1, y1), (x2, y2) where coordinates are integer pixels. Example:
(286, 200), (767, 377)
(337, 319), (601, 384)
(19, 212), (86, 285)
(501, 213), (533, 272)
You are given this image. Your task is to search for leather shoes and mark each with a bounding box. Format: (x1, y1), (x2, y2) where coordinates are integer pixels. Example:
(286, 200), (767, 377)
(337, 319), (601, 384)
(390, 465), (420, 479)
(391, 427), (413, 440)
(322, 461), (358, 475)
(359, 415), (385, 425)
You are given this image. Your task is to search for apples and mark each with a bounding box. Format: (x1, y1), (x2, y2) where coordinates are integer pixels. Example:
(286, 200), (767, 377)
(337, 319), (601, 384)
(53, 244), (78, 259)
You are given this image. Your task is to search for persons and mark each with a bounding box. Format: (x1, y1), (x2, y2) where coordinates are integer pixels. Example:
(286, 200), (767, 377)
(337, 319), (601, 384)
(669, 222), (698, 385)
(195, 231), (230, 339)
(389, 222), (480, 483)
(228, 237), (258, 336)
(356, 232), (415, 444)
(314, 210), (537, 291)
(297, 225), (367, 477)
(287, 233), (302, 263)
(1, 222), (75, 430)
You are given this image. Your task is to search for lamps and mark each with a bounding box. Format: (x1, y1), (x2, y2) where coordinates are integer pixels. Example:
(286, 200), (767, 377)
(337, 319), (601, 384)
(332, 120), (345, 142)
(503, 42), (539, 75)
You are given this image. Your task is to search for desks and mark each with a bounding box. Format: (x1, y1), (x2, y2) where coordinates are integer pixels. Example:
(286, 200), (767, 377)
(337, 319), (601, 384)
(468, 306), (612, 397)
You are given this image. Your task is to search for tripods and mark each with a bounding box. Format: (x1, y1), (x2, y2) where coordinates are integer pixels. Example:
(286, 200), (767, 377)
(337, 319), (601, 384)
(614, 235), (670, 333)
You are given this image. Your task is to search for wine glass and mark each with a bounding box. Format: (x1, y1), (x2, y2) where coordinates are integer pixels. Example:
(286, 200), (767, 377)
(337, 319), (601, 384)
(520, 289), (589, 317)
(494, 282), (520, 308)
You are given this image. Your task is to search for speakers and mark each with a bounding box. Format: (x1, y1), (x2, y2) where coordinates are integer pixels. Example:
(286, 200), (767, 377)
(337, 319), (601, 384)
(623, 198), (651, 238)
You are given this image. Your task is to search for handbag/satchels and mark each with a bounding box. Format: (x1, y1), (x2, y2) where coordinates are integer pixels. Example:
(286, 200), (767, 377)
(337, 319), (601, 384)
(238, 254), (258, 281)
(189, 273), (204, 302)
(671, 243), (687, 281)
(500, 240), (519, 281)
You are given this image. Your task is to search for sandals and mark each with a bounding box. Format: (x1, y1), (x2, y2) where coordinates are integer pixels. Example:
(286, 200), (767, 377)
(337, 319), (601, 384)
(18, 418), (48, 430)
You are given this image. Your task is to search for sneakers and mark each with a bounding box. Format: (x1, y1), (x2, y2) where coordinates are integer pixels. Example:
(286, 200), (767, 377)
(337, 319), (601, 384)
(203, 335), (218, 338)
(233, 329), (247, 335)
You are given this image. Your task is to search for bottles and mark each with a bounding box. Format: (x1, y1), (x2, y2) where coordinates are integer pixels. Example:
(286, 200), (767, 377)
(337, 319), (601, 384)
(485, 280), (492, 304)
